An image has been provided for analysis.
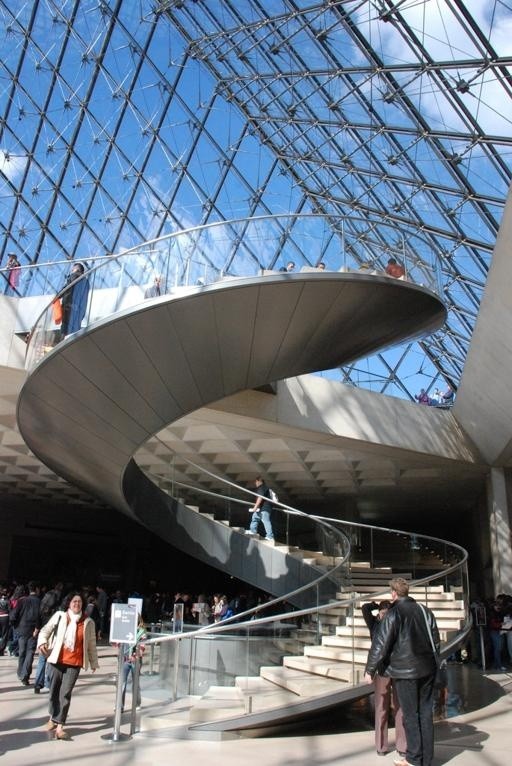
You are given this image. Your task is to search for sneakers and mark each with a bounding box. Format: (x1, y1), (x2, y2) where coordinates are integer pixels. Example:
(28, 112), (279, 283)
(48, 718), (69, 739)
(113, 706), (142, 712)
(22, 676), (41, 693)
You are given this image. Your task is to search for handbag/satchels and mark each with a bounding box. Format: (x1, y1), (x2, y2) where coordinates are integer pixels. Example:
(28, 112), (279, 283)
(221, 609), (234, 623)
(434, 657), (448, 690)
(269, 489), (278, 502)
(39, 629), (56, 658)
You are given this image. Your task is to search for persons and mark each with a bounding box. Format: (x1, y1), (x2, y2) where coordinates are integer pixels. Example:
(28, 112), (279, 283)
(10, 582), (100, 740)
(4, 253), (21, 297)
(317, 263), (325, 272)
(144, 276), (171, 300)
(244, 476), (275, 545)
(414, 385), (454, 406)
(279, 267), (289, 277)
(364, 577), (440, 766)
(52, 262), (90, 341)
(362, 600), (408, 757)
(339, 258), (404, 281)
(0, 578), (258, 657)
(287, 261), (295, 272)
(446, 594), (512, 672)
(110, 612), (146, 713)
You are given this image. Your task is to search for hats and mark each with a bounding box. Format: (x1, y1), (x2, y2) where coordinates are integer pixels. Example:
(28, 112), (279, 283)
(7, 253), (17, 257)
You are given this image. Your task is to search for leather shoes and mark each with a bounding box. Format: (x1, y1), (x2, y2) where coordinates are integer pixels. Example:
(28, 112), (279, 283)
(377, 748), (414, 766)
